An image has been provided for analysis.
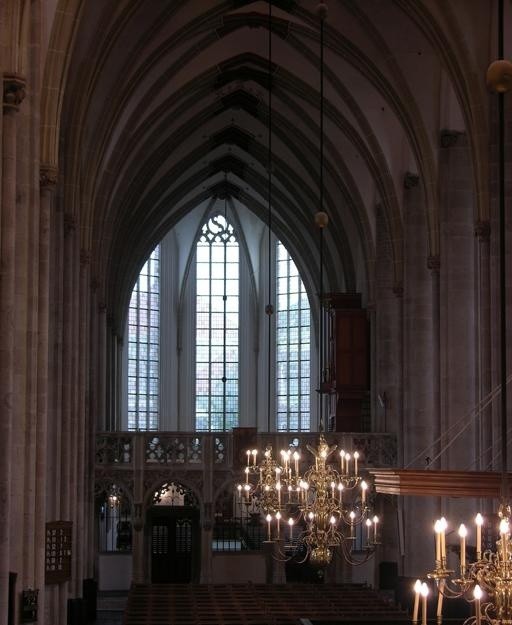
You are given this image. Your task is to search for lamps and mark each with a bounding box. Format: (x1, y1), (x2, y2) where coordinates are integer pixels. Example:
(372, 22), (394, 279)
(411, 0), (512, 625)
(264, 3), (378, 568)
(236, 1), (301, 525)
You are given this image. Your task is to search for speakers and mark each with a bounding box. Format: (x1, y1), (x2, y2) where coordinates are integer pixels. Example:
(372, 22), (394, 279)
(68, 598), (87, 625)
(378, 562), (398, 589)
(83, 578), (97, 625)
